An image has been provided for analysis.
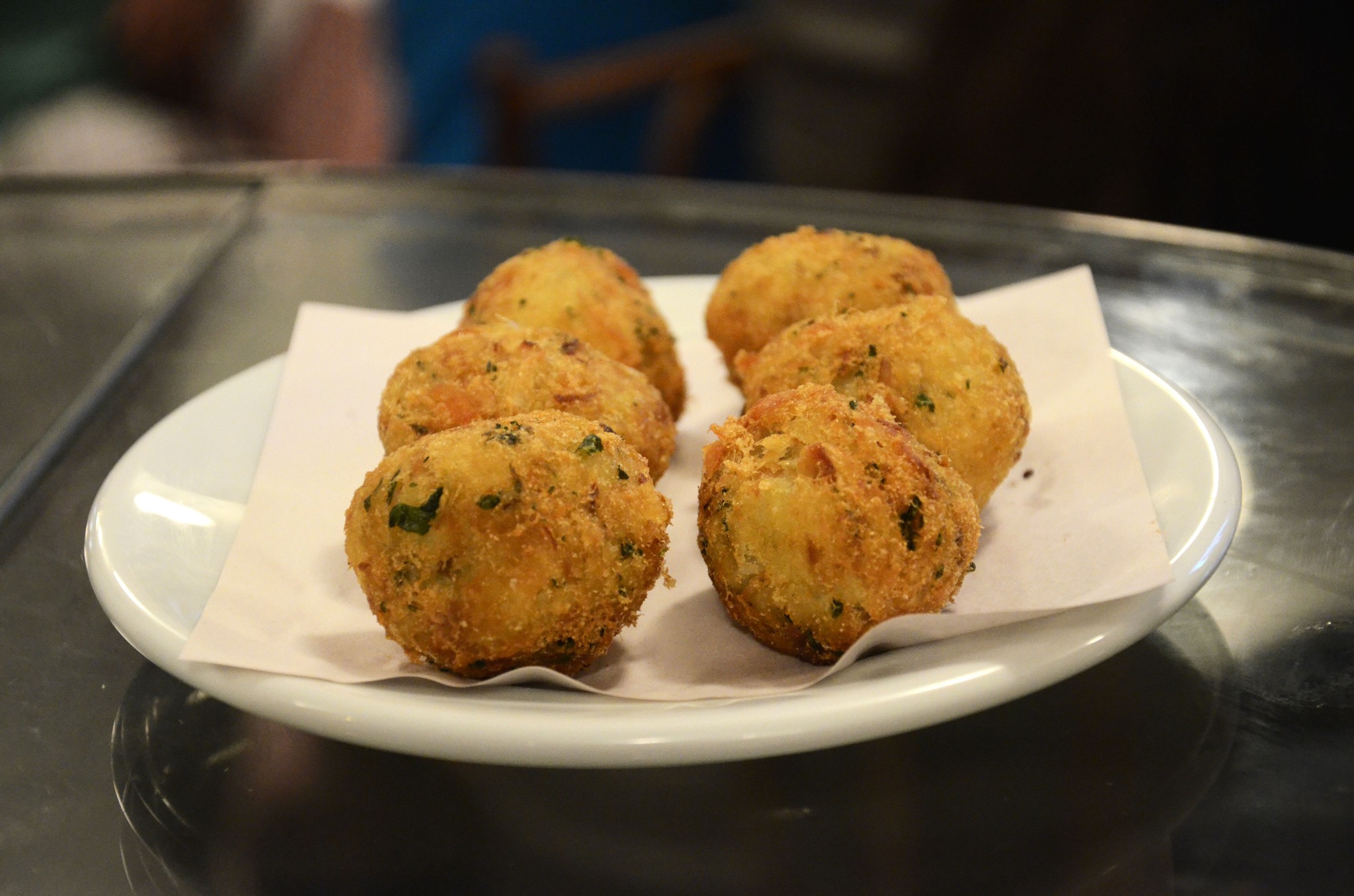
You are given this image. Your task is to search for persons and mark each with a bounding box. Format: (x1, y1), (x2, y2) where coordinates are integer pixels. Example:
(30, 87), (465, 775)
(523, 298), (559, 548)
(1, 0), (759, 182)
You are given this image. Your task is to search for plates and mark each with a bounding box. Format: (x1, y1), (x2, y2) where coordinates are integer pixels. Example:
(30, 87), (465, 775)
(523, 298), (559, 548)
(82, 266), (1237, 768)
